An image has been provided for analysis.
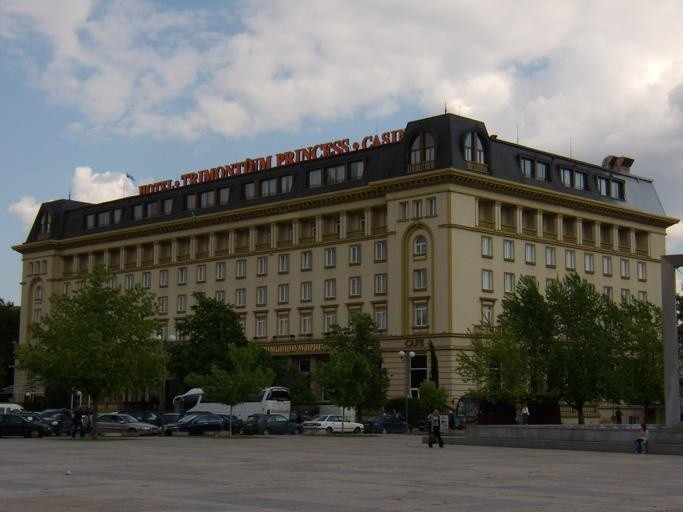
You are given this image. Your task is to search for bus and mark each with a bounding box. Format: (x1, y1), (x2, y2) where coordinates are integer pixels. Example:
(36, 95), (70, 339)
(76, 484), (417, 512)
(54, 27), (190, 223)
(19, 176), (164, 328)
(170, 384), (292, 422)
(450, 390), (562, 429)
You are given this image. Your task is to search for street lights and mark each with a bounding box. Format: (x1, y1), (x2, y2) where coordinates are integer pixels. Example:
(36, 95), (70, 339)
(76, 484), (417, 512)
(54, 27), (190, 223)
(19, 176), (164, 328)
(397, 345), (417, 435)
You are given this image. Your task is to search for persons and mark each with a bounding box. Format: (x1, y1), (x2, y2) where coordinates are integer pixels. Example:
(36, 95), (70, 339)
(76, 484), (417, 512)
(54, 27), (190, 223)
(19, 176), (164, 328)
(632, 422), (649, 454)
(7, 406), (92, 440)
(426, 408), (444, 448)
(615, 407), (623, 423)
(521, 402), (530, 425)
